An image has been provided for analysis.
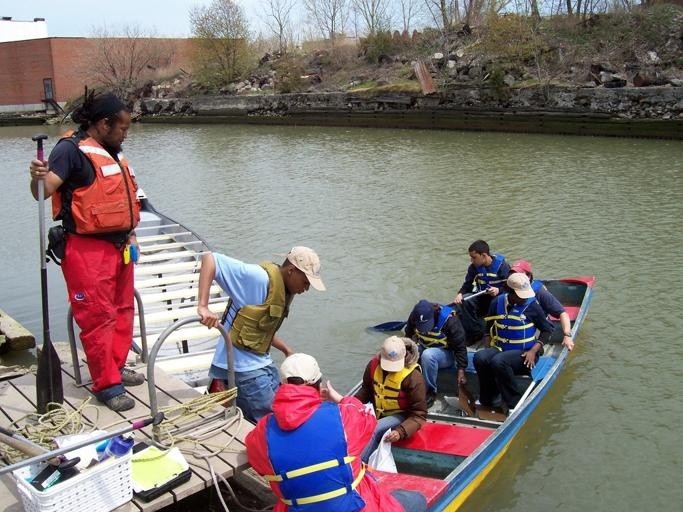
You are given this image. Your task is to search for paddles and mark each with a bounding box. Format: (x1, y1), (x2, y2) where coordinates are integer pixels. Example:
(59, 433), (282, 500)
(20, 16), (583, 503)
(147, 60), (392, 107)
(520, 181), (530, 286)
(375, 285), (489, 332)
(504, 356), (556, 421)
(31, 135), (63, 416)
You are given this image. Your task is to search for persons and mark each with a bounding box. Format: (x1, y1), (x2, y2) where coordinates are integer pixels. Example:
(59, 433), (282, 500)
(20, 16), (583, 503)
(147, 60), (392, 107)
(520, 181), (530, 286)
(455, 239), (510, 341)
(406, 298), (468, 407)
(352, 335), (427, 471)
(470, 271), (555, 416)
(508, 260), (575, 352)
(244, 352), (427, 512)
(198, 244), (329, 424)
(28, 92), (149, 413)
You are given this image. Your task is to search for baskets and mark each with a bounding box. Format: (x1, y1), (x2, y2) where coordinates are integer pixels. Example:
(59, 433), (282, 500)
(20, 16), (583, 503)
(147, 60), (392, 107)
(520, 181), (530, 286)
(13, 432), (132, 512)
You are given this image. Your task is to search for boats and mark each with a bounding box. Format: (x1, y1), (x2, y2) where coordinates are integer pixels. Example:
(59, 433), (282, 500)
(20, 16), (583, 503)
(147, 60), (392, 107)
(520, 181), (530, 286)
(248, 268), (597, 507)
(127, 186), (230, 397)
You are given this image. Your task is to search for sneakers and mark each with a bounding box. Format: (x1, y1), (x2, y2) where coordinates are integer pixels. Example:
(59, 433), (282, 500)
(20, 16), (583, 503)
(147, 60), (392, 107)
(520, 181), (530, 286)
(105, 393), (134, 411)
(120, 367), (146, 385)
(426, 392), (435, 408)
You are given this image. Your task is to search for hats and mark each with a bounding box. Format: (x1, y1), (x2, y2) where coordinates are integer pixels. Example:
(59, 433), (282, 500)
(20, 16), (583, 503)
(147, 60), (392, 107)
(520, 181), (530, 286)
(413, 299), (435, 332)
(380, 335), (406, 372)
(287, 245), (326, 292)
(509, 259), (532, 273)
(279, 353), (322, 386)
(507, 272), (536, 299)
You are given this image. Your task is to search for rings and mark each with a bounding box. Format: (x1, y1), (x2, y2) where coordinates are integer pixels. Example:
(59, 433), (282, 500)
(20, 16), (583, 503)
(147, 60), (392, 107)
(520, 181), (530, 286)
(35, 170), (39, 175)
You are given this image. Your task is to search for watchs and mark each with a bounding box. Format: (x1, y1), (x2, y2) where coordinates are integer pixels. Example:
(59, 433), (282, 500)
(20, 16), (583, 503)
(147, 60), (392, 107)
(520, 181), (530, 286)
(563, 332), (572, 337)
